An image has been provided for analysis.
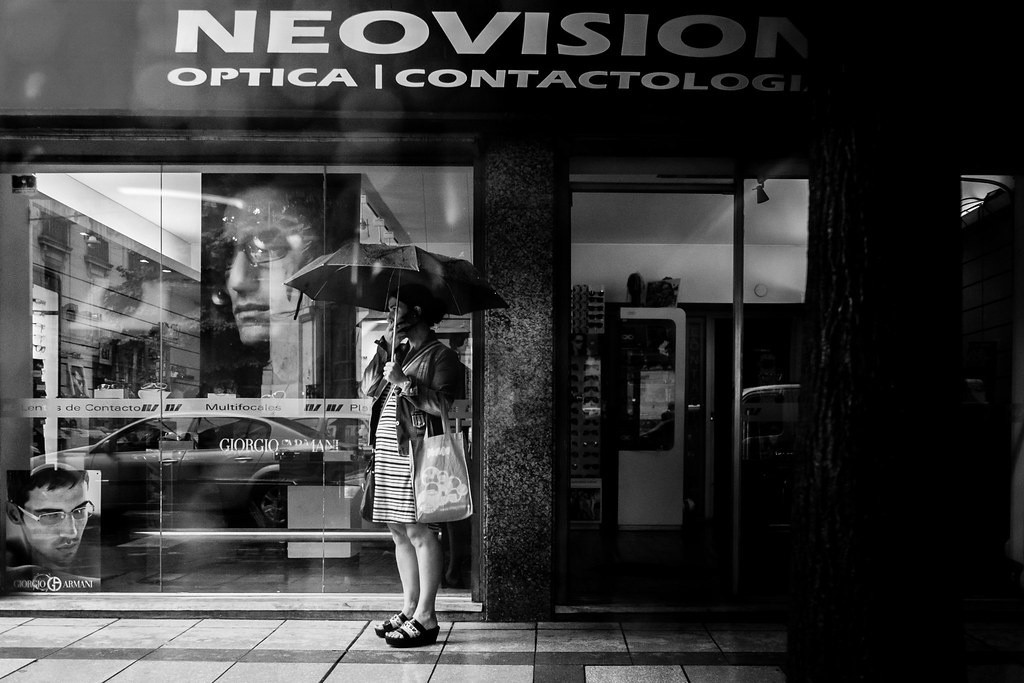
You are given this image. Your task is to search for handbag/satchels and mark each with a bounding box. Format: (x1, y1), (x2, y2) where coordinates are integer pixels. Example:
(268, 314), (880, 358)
(408, 389), (474, 523)
(358, 453), (386, 528)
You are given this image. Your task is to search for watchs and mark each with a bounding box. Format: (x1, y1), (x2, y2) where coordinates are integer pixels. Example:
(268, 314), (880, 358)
(402, 380), (410, 391)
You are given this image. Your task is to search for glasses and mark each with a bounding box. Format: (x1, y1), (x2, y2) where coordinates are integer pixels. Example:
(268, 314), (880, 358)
(10, 500), (95, 525)
(205, 229), (330, 273)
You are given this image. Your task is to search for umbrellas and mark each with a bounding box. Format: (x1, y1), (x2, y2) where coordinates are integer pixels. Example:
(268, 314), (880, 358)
(286, 241), (509, 363)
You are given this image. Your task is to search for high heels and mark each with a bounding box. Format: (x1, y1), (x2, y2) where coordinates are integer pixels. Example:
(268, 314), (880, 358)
(384, 616), (440, 648)
(374, 611), (413, 638)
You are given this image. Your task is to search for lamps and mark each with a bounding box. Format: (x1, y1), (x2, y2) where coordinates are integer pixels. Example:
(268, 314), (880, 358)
(753, 177), (769, 205)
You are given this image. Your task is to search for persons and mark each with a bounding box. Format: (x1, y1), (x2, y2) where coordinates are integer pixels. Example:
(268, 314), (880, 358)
(359, 282), (461, 647)
(205, 178), (355, 398)
(5, 471), (101, 591)
